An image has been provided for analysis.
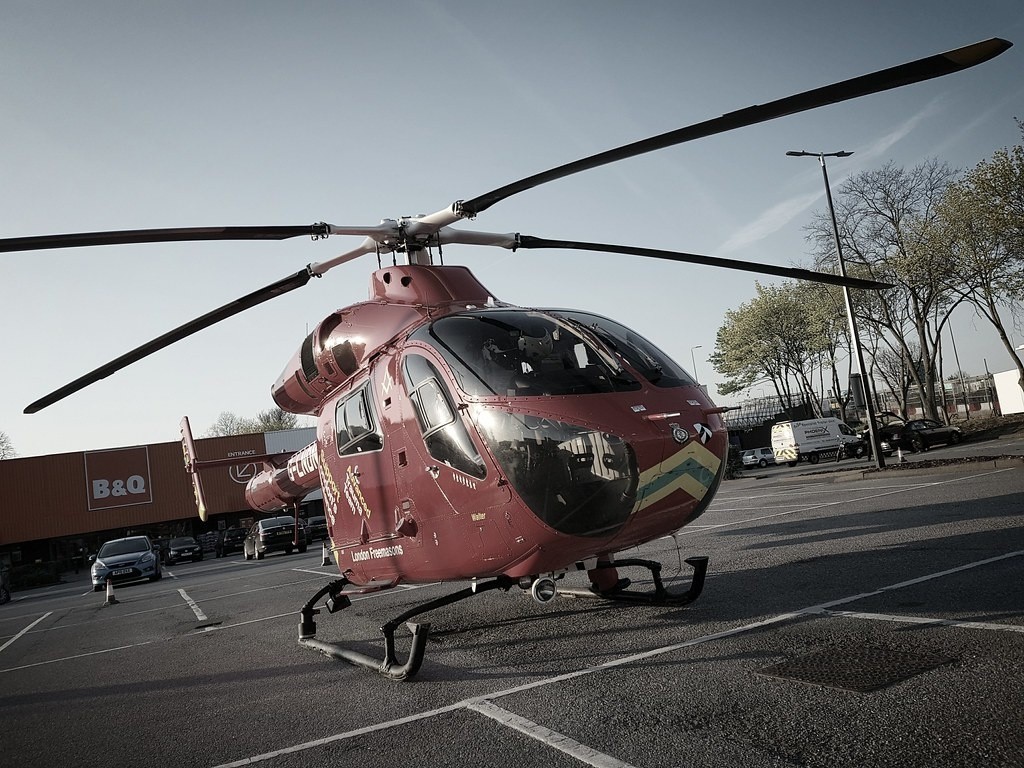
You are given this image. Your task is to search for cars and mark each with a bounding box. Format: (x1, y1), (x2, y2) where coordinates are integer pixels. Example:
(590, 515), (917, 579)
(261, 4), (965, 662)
(88, 535), (162, 592)
(875, 412), (962, 458)
(294, 518), (313, 545)
(305, 516), (329, 540)
(0, 561), (11, 605)
(214, 527), (253, 558)
(242, 516), (307, 560)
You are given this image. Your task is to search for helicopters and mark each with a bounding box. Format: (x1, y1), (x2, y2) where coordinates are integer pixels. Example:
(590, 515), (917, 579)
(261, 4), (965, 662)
(0, 34), (1017, 683)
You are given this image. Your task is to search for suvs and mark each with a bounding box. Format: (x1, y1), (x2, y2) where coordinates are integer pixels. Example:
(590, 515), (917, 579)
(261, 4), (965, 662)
(742, 447), (775, 470)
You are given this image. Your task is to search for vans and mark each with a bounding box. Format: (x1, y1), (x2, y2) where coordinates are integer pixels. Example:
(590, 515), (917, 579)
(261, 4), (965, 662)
(771, 417), (863, 467)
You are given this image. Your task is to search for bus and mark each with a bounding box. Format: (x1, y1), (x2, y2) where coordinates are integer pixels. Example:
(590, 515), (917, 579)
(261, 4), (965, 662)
(163, 536), (203, 565)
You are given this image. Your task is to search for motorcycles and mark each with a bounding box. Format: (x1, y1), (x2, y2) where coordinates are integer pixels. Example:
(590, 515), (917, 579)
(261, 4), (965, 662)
(835, 434), (864, 462)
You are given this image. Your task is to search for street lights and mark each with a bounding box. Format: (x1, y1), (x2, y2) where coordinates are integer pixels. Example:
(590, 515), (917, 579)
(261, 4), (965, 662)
(785, 149), (888, 472)
(940, 309), (966, 393)
(690, 345), (702, 383)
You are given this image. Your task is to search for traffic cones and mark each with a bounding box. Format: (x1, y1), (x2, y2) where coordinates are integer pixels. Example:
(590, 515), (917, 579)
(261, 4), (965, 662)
(103, 577), (119, 607)
(321, 543), (333, 566)
